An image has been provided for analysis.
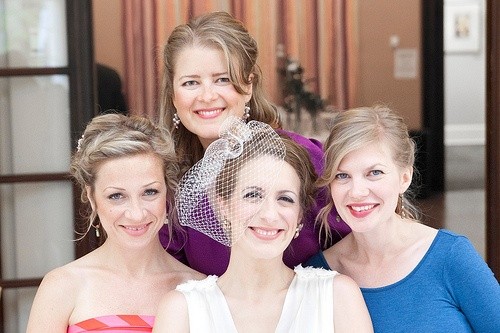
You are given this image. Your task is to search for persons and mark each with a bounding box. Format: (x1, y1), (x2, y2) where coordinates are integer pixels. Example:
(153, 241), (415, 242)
(152, 11), (352, 276)
(152, 113), (375, 333)
(304, 104), (500, 333)
(26, 112), (210, 333)
(97, 62), (128, 117)
